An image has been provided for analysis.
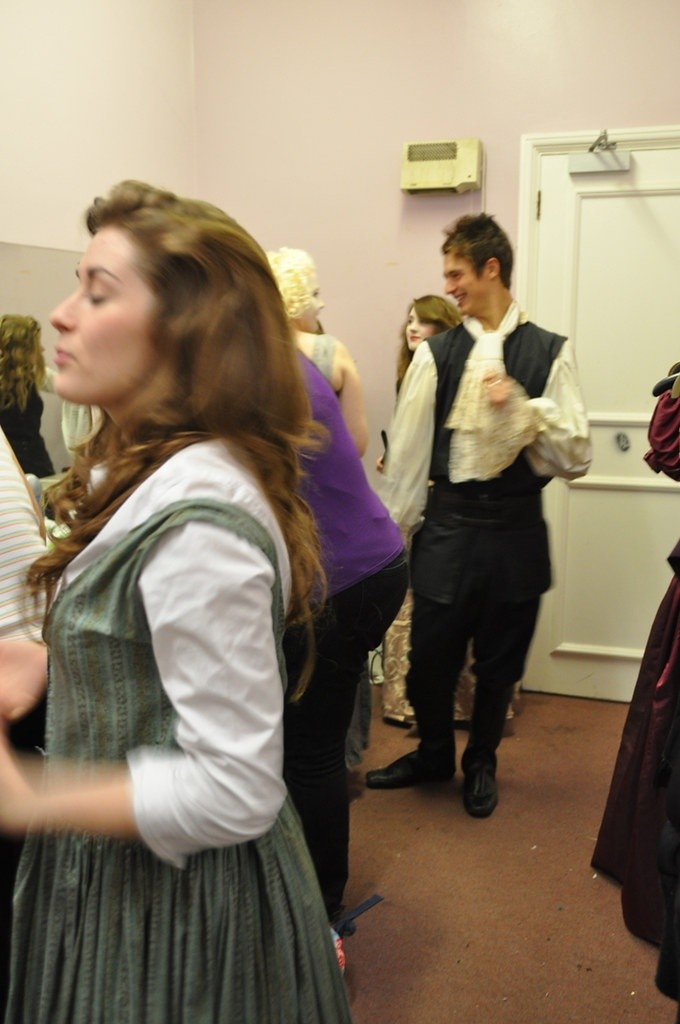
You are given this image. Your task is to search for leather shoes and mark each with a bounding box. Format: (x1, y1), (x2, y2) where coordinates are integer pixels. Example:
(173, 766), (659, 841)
(366, 749), (457, 790)
(463, 760), (498, 818)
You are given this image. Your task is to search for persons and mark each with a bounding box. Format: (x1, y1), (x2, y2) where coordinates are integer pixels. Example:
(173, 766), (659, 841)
(0, 243), (521, 803)
(0, 179), (356, 1024)
(589, 364), (680, 1005)
(381, 213), (595, 818)
